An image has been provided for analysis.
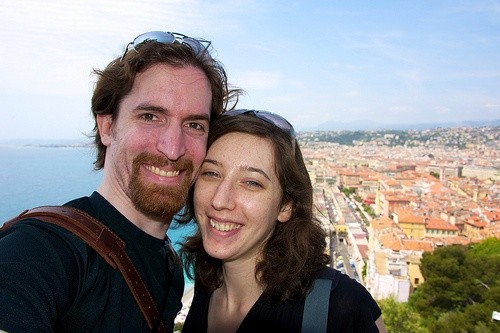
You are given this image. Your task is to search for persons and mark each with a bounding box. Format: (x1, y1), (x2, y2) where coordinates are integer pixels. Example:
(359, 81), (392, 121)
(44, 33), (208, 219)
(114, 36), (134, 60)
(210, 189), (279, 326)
(0, 38), (248, 333)
(178, 109), (386, 333)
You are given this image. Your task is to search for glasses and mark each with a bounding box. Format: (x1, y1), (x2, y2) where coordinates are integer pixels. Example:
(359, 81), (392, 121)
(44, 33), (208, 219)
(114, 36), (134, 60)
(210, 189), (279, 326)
(120, 30), (209, 68)
(212, 108), (296, 163)
